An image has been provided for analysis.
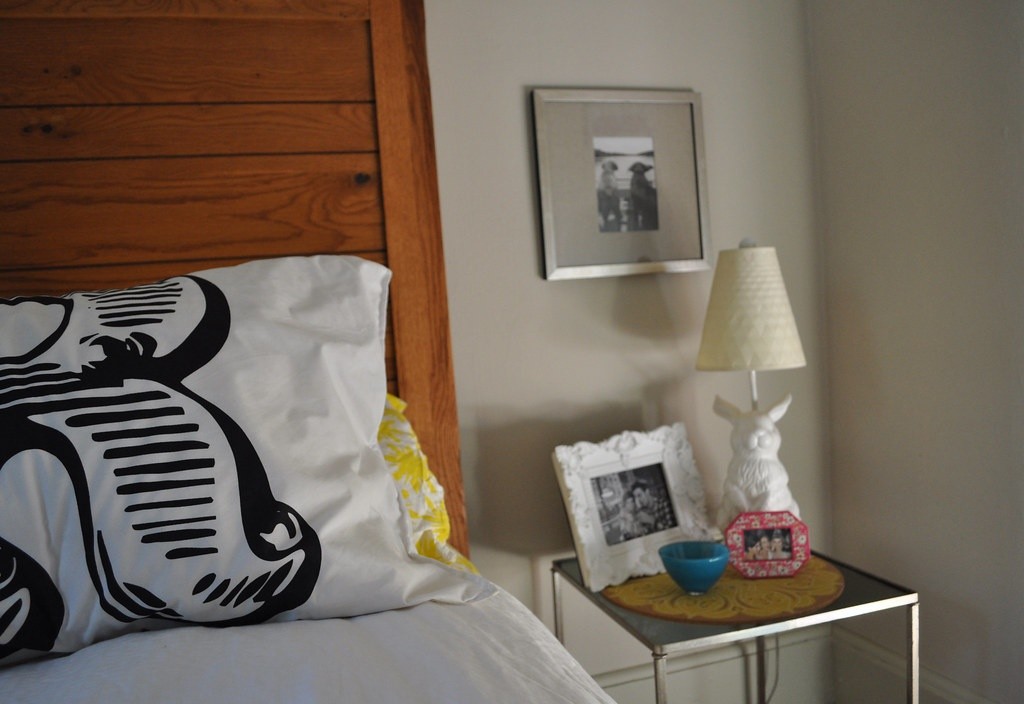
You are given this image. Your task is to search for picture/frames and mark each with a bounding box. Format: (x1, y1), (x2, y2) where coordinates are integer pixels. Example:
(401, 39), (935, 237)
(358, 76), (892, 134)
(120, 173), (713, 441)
(723, 511), (810, 579)
(532, 87), (715, 284)
(550, 421), (708, 594)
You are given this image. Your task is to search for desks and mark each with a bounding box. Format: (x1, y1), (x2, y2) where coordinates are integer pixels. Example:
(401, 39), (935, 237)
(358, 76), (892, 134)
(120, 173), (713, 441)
(549, 548), (921, 704)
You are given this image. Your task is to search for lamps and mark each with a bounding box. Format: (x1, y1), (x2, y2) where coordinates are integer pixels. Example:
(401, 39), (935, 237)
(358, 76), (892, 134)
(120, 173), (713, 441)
(694, 246), (808, 534)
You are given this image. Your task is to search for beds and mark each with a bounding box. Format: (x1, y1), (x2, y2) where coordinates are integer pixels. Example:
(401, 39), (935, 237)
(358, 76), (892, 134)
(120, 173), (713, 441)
(0, 0), (613, 704)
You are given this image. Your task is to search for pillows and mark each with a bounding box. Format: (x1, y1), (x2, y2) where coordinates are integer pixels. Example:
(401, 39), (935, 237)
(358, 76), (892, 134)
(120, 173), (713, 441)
(0, 253), (501, 671)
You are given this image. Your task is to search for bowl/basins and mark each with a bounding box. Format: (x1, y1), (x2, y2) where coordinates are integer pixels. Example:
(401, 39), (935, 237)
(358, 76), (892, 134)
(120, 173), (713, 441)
(658, 542), (730, 595)
